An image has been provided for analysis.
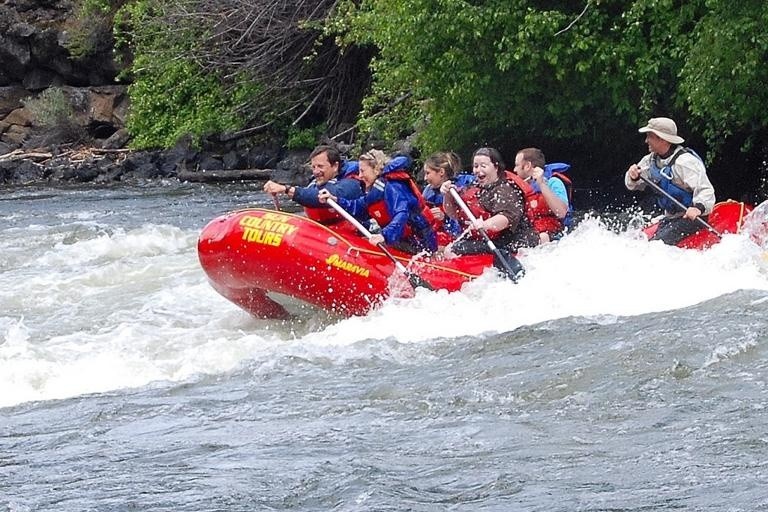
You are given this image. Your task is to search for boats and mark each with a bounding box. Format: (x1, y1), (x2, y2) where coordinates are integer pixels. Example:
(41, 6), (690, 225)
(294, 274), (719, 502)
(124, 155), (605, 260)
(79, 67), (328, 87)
(192, 198), (763, 340)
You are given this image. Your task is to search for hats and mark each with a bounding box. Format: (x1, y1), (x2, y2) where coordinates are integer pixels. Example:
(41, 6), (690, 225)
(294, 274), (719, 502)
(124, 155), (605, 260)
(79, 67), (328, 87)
(637, 116), (686, 146)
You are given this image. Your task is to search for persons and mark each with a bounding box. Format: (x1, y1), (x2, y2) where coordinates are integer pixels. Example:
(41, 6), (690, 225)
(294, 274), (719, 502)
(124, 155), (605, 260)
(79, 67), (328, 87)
(624, 116), (716, 246)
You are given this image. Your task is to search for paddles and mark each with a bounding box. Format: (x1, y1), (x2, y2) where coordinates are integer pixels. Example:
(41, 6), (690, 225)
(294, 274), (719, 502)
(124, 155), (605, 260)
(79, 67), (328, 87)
(450, 181), (526, 282)
(322, 191), (435, 292)
